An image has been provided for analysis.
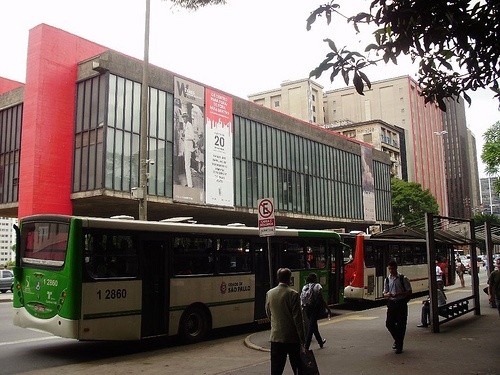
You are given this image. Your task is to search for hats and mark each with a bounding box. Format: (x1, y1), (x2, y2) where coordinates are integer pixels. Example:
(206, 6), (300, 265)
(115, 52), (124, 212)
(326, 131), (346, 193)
(493, 257), (500, 266)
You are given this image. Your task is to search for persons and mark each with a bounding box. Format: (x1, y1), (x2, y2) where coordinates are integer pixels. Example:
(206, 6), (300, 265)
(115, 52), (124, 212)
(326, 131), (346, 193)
(417, 280), (446, 327)
(383, 261), (413, 355)
(435, 254), (499, 312)
(90, 234), (256, 276)
(299, 274), (331, 349)
(180, 112), (198, 189)
(265, 268), (306, 374)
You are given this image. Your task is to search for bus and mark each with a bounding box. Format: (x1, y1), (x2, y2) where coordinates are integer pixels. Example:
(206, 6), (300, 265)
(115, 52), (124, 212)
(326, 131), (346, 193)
(337, 230), (457, 301)
(12, 213), (346, 346)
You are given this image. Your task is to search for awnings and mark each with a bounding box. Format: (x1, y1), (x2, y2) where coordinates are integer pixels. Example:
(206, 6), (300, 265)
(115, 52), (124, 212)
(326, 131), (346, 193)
(370, 217), (472, 245)
(474, 222), (499, 248)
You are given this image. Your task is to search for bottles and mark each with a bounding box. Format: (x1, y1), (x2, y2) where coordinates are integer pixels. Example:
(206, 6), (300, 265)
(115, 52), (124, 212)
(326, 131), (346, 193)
(327, 312), (331, 320)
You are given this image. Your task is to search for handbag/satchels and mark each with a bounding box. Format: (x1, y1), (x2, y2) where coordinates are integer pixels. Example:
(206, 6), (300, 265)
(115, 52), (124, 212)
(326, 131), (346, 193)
(302, 283), (324, 307)
(298, 345), (320, 375)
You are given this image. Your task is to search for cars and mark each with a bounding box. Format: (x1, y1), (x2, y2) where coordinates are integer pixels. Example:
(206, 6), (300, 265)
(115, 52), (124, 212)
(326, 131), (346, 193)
(455, 254), (486, 275)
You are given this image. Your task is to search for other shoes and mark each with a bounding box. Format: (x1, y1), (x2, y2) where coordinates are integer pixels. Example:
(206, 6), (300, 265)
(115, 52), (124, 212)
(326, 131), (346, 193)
(319, 339), (326, 348)
(392, 341), (397, 349)
(417, 324), (428, 327)
(395, 347), (404, 354)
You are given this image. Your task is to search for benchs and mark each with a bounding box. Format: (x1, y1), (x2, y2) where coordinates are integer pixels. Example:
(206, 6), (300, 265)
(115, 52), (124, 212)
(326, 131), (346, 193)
(437, 299), (468, 318)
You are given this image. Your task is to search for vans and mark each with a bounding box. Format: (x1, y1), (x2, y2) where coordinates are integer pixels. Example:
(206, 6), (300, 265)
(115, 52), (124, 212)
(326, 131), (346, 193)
(0, 270), (14, 293)
